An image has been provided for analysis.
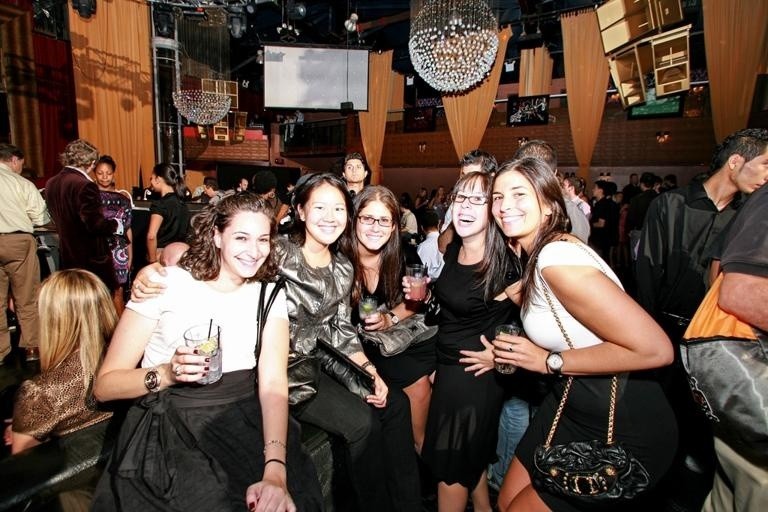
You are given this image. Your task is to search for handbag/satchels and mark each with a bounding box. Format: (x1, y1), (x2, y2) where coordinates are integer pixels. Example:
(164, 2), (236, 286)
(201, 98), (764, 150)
(310, 337), (376, 406)
(254, 339), (323, 417)
(533, 439), (657, 504)
(356, 287), (440, 358)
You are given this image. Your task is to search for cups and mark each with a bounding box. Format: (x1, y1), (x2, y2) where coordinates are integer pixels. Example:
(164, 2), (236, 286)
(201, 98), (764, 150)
(403, 262), (429, 301)
(185, 323), (224, 384)
(494, 322), (521, 374)
(357, 297), (378, 333)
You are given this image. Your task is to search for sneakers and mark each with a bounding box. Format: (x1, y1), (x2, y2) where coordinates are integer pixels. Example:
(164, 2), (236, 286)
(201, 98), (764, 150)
(25, 347), (40, 362)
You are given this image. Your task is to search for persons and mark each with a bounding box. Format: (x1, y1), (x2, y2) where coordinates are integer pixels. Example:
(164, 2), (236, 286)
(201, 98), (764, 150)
(45, 139), (123, 296)
(699, 181), (768, 512)
(401, 172), (522, 512)
(341, 185), (431, 458)
(4, 268), (120, 511)
(637, 128), (768, 336)
(265, 181), (296, 223)
(147, 164), (249, 263)
(460, 155), (674, 512)
(227, 15), (246, 39)
(517, 140), (677, 274)
(343, 153), (368, 192)
(0, 143), (54, 365)
(400, 150), (498, 280)
(130, 173), (421, 512)
(95, 193), (324, 512)
(90, 155), (135, 318)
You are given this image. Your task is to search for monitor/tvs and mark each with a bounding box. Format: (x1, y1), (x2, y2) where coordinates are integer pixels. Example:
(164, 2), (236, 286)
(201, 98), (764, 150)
(403, 106), (436, 133)
(506, 95), (550, 127)
(627, 92), (687, 120)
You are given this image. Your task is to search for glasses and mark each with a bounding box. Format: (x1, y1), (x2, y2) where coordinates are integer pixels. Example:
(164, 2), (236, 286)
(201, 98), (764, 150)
(450, 192), (491, 206)
(356, 215), (395, 228)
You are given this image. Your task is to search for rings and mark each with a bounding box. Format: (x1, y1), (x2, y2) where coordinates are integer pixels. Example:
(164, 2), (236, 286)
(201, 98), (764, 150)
(508, 344), (514, 354)
(136, 283), (141, 290)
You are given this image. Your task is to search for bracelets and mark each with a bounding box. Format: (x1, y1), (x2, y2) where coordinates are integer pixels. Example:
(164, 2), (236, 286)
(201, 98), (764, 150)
(546, 354), (551, 377)
(261, 459), (286, 468)
(262, 440), (286, 453)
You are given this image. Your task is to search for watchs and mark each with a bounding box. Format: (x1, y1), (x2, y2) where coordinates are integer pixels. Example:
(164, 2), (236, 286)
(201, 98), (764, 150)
(388, 312), (399, 325)
(547, 350), (565, 380)
(146, 362), (164, 395)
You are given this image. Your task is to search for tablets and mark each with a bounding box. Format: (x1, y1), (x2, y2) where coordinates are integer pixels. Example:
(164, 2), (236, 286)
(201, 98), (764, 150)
(315, 335), (376, 395)
